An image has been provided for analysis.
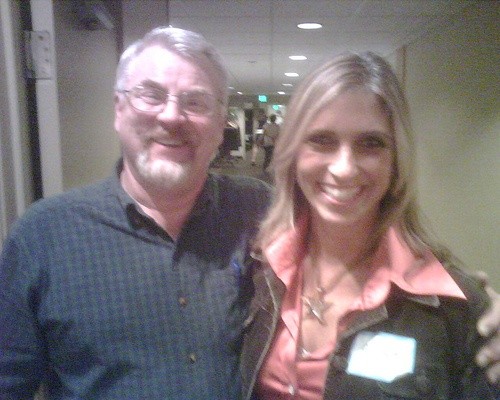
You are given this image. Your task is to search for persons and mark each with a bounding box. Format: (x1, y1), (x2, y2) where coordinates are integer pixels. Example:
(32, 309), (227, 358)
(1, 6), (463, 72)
(236, 49), (499, 400)
(258, 114), (280, 174)
(250, 107), (268, 165)
(1, 23), (499, 400)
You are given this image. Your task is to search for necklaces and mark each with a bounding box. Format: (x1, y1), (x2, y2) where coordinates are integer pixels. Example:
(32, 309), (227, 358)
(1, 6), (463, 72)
(299, 226), (377, 328)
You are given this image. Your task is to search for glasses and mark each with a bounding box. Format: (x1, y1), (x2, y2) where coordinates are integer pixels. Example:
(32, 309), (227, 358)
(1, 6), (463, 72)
(115, 85), (225, 115)
